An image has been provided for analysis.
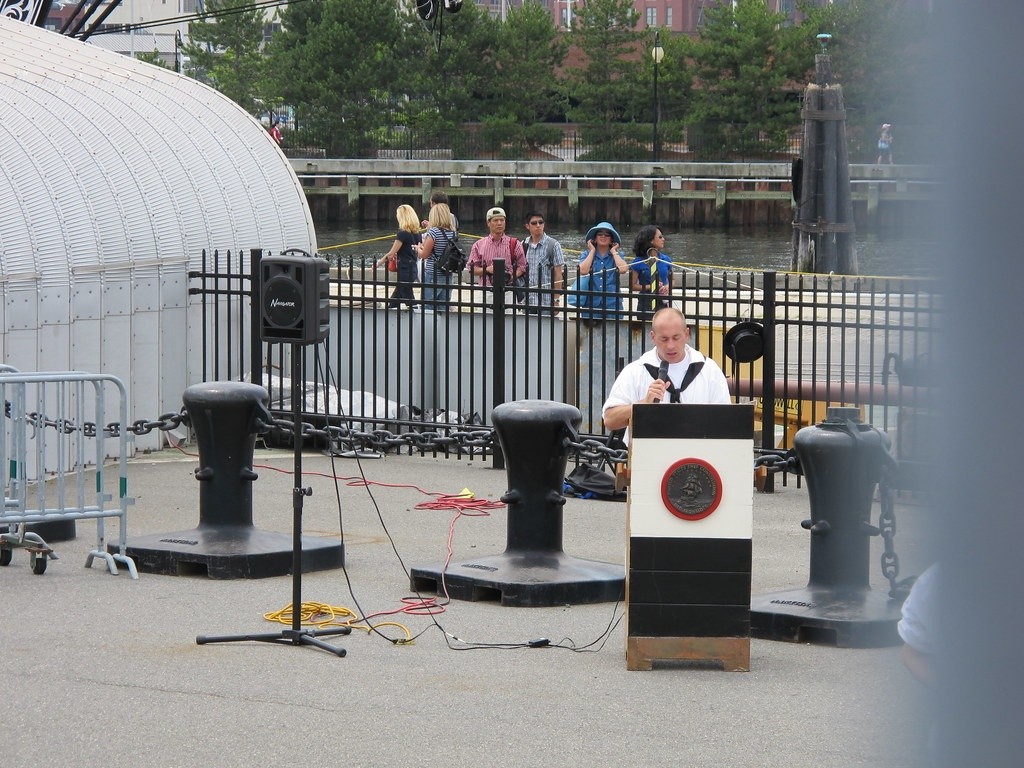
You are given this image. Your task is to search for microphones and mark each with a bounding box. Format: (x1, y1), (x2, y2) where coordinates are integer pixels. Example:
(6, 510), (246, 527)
(653, 360), (669, 403)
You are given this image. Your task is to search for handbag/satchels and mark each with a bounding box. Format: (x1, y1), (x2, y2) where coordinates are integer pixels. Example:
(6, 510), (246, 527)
(387, 253), (398, 272)
(878, 139), (889, 150)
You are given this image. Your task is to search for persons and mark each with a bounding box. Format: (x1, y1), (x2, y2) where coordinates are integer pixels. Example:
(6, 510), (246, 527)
(877, 122), (895, 165)
(466, 206), (526, 310)
(421, 192), (460, 232)
(412, 203), (460, 311)
(567, 221), (628, 319)
(601, 307), (733, 448)
(630, 225), (675, 320)
(372, 203), (423, 309)
(270, 120), (282, 145)
(517, 211), (563, 316)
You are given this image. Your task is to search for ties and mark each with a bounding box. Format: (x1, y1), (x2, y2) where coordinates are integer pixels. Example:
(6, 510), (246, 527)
(645, 360), (706, 404)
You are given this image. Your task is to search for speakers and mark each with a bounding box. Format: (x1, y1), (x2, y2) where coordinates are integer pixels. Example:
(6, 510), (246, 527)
(258, 248), (331, 346)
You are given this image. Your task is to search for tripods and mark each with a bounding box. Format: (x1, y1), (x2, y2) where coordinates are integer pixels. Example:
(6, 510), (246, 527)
(197, 348), (352, 660)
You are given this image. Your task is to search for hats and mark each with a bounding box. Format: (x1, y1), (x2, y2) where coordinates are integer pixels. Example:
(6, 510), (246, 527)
(585, 222), (621, 248)
(486, 206), (507, 222)
(881, 123), (891, 128)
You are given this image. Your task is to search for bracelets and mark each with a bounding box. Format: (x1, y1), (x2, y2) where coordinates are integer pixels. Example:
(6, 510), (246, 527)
(613, 252), (619, 256)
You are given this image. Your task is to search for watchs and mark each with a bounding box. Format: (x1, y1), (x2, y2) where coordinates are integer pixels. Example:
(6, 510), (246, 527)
(554, 298), (560, 302)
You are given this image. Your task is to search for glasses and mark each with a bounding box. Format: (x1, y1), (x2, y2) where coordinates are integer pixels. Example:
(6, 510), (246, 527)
(528, 220), (545, 225)
(653, 235), (664, 240)
(594, 231), (612, 237)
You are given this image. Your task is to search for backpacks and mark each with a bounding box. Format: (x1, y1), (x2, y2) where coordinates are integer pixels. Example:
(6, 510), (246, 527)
(434, 227), (469, 274)
